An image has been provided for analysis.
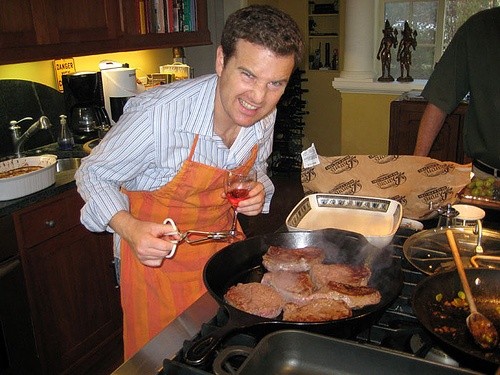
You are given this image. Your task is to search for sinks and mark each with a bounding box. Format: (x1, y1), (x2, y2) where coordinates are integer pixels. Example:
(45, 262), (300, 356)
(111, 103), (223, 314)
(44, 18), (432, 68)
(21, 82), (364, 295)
(56, 157), (84, 174)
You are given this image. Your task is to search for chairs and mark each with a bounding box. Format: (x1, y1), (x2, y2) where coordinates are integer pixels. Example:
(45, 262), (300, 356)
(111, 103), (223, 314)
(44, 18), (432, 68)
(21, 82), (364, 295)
(0, 80), (63, 158)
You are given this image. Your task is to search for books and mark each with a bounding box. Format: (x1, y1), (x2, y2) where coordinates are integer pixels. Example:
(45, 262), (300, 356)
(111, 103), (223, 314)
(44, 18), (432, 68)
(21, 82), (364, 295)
(144, 65), (194, 90)
(145, 0), (197, 33)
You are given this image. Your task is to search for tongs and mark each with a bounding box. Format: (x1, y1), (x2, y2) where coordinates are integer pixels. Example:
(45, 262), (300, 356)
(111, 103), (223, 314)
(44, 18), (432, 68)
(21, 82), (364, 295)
(161, 217), (227, 258)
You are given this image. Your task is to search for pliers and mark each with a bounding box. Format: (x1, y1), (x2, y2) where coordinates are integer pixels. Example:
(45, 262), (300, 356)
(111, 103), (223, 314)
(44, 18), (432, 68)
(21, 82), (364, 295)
(162, 218), (238, 258)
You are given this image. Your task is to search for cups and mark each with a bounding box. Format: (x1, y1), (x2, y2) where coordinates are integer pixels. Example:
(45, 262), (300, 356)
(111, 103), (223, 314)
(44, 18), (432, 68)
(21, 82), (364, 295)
(450, 204), (485, 226)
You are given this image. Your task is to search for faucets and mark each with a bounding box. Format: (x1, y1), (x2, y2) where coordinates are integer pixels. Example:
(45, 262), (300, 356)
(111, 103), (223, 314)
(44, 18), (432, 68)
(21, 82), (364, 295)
(9, 116), (52, 157)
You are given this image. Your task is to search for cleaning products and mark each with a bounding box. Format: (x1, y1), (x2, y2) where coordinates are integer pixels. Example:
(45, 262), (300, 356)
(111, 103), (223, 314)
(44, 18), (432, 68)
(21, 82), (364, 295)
(57, 114), (74, 150)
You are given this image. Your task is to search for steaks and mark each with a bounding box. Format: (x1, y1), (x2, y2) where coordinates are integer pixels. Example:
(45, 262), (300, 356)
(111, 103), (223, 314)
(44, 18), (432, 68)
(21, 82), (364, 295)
(224, 245), (381, 323)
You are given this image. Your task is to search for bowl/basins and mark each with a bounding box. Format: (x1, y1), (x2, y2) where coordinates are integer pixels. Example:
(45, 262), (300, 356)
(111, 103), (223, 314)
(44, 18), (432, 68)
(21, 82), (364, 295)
(0, 156), (57, 202)
(286, 193), (402, 250)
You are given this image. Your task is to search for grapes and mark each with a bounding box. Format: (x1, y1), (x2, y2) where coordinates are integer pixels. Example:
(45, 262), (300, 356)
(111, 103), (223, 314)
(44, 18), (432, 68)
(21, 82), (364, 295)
(466, 176), (496, 197)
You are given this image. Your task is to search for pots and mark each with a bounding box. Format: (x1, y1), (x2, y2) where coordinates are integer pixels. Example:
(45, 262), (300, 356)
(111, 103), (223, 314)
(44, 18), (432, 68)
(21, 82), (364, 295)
(414, 268), (500, 365)
(184, 228), (401, 364)
(212, 330), (484, 375)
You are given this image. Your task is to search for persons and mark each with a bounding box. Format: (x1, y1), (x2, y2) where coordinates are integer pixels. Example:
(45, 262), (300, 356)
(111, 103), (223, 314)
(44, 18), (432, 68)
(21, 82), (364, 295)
(377, 19), (417, 78)
(414, 6), (500, 178)
(74, 4), (303, 360)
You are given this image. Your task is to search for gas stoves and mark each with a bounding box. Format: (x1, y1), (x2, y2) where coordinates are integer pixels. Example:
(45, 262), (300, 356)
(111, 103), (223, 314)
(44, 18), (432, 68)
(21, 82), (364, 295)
(108, 226), (500, 375)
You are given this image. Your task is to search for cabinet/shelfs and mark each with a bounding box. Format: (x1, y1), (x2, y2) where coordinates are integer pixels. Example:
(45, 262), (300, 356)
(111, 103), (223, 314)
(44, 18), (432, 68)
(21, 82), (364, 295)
(389, 90), (474, 165)
(0, 0), (214, 65)
(305, 0), (344, 72)
(0, 188), (123, 375)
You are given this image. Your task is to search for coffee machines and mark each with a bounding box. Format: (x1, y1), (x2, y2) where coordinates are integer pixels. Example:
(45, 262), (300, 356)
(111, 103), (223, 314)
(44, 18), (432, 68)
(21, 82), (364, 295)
(62, 71), (113, 144)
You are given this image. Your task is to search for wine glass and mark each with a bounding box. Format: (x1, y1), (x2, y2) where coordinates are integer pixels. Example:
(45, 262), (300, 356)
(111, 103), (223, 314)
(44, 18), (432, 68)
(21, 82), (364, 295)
(223, 163), (258, 244)
